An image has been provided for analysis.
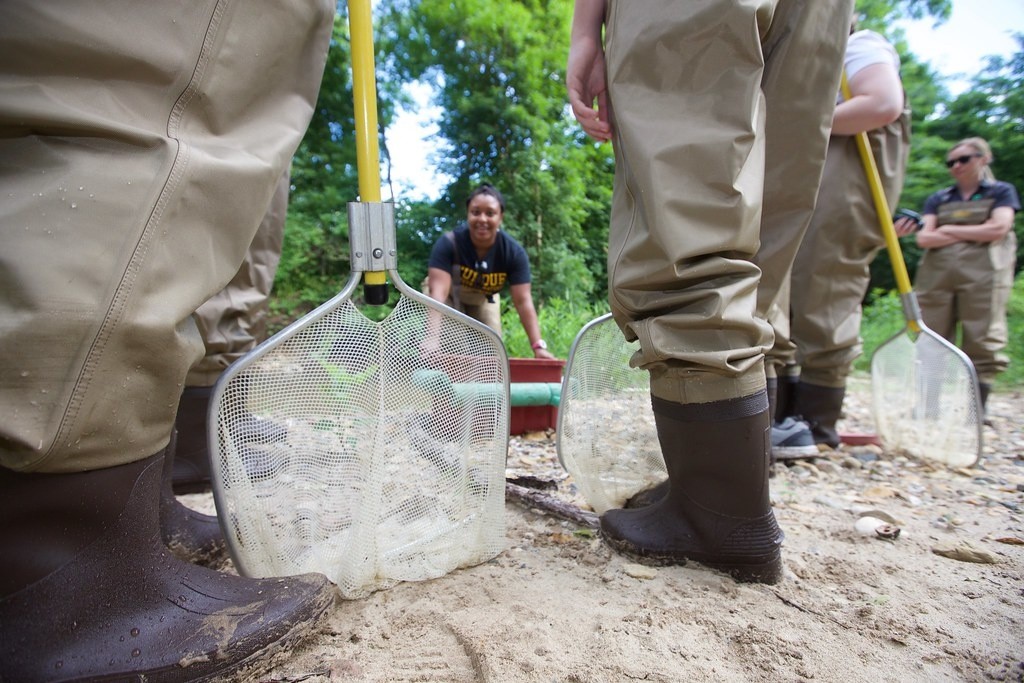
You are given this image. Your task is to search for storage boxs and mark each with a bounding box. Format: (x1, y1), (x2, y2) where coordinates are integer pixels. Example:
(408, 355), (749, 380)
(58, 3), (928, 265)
(431, 355), (566, 440)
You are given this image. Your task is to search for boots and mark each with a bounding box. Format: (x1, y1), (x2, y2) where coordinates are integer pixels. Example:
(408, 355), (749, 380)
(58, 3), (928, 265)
(967, 381), (991, 424)
(0, 429), (338, 683)
(170, 375), (288, 497)
(790, 381), (845, 449)
(908, 377), (942, 420)
(597, 388), (784, 585)
(766, 376), (799, 428)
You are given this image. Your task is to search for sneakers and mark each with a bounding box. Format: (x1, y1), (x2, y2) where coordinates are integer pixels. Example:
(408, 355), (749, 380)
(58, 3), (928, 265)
(769, 413), (820, 459)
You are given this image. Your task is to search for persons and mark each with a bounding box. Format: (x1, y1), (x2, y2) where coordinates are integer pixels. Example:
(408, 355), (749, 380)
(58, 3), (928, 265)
(772, 12), (912, 458)
(1, 0), (336, 683)
(914, 138), (1022, 427)
(417, 183), (555, 360)
(893, 216), (919, 240)
(566, 0), (855, 585)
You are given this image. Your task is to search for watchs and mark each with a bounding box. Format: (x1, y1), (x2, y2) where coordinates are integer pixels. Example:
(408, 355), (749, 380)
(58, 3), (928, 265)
(530, 340), (547, 350)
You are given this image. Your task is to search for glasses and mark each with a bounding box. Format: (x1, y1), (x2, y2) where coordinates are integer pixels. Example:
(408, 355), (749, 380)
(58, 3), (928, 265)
(944, 152), (982, 168)
